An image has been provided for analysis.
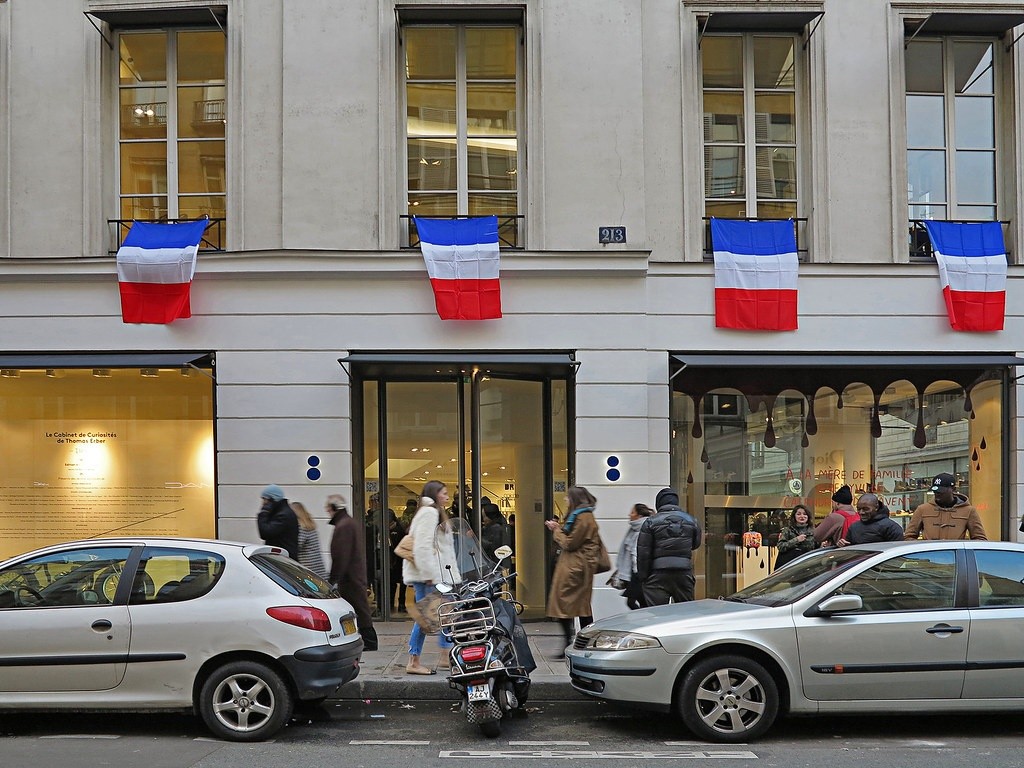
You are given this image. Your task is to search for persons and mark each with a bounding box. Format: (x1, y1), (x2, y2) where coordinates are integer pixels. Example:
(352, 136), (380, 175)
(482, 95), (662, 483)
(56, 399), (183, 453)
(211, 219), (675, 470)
(323, 495), (378, 651)
(904, 473), (988, 542)
(289, 502), (330, 582)
(453, 495), (561, 604)
(545, 487), (599, 661)
(751, 513), (767, 532)
(814, 484), (904, 548)
(256, 484), (298, 562)
(402, 480), (462, 675)
(617, 504), (657, 611)
(637, 488), (702, 607)
(363, 492), (417, 614)
(774, 505), (825, 573)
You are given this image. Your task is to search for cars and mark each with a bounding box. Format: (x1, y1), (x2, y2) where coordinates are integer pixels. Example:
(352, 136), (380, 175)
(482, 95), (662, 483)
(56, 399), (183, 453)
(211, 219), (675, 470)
(564, 543), (1024, 744)
(0, 538), (365, 741)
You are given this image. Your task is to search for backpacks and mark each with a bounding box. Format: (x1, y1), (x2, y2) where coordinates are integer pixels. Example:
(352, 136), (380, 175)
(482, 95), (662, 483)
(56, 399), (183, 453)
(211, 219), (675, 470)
(834, 510), (861, 539)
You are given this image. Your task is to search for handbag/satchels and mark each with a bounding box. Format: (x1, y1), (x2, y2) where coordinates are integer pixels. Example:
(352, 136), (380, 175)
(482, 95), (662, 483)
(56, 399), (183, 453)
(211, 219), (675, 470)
(409, 589), (461, 633)
(393, 535), (415, 563)
(774, 526), (810, 571)
(596, 539), (612, 574)
(606, 570), (626, 590)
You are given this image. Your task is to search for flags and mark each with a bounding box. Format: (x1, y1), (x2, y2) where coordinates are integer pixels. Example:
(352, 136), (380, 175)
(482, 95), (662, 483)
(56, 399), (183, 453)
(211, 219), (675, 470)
(116, 219), (209, 323)
(708, 218), (798, 331)
(920, 219), (1007, 332)
(410, 216), (503, 320)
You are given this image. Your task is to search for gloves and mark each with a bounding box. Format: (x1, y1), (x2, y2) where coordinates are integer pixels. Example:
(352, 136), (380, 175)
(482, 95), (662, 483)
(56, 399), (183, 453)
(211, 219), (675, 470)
(262, 501), (274, 512)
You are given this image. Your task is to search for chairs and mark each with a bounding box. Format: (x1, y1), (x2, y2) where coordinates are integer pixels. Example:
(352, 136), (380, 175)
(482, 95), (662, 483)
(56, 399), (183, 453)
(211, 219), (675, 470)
(181, 574), (198, 583)
(888, 569), (920, 609)
(154, 580), (181, 599)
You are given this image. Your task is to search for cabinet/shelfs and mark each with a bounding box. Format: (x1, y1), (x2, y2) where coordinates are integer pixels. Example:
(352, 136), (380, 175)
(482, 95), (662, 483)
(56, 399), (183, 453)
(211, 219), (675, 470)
(878, 476), (969, 530)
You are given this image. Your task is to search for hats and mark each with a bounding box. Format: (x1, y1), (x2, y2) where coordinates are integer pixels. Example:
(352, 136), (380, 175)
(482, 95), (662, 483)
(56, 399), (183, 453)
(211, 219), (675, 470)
(656, 489), (679, 511)
(928, 473), (956, 491)
(832, 485), (852, 504)
(260, 486), (284, 502)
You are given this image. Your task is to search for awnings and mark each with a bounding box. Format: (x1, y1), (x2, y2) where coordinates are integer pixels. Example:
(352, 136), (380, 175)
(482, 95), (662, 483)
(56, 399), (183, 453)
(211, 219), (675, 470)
(697, 11), (825, 50)
(905, 11), (1024, 52)
(669, 353), (1024, 381)
(83, 4), (229, 51)
(393, 4), (527, 46)
(0, 353), (215, 379)
(336, 353), (582, 380)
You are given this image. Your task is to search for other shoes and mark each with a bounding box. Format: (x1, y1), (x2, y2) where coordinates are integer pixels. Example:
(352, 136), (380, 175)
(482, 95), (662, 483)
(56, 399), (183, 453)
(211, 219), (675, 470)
(407, 664), (436, 675)
(550, 653), (565, 662)
(397, 607), (407, 613)
(437, 658), (450, 669)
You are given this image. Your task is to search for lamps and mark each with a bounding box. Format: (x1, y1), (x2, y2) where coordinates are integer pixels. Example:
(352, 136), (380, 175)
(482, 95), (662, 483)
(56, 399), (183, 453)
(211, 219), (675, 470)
(1, 370), (20, 379)
(140, 368), (159, 378)
(180, 368), (190, 378)
(45, 370), (52, 376)
(92, 368), (112, 379)
(50, 368), (57, 378)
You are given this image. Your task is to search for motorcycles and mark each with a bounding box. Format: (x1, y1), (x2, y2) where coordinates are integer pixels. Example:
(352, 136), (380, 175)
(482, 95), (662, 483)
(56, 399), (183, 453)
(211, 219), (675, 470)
(431, 516), (538, 739)
